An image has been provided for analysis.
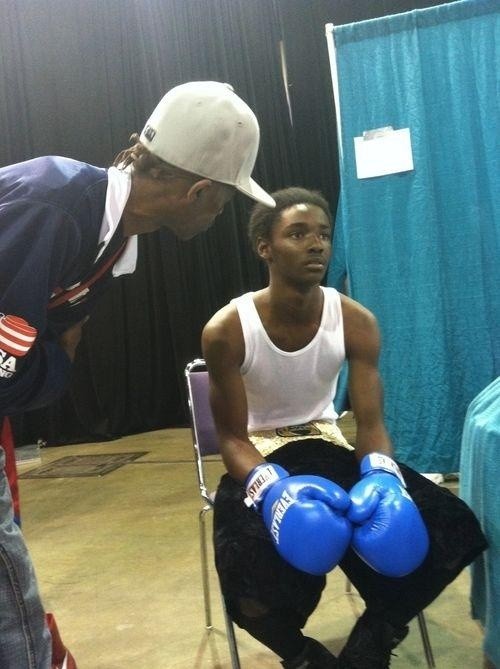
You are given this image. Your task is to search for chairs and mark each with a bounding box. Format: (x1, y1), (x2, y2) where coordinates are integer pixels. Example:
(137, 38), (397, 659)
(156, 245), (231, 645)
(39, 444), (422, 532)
(184, 358), (436, 669)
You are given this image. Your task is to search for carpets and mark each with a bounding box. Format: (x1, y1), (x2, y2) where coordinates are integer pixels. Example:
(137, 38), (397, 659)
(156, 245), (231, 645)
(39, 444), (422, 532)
(16, 450), (150, 477)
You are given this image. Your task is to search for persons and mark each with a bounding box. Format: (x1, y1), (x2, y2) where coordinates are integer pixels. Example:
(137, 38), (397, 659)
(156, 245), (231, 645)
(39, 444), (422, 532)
(1, 77), (279, 667)
(195, 185), (493, 668)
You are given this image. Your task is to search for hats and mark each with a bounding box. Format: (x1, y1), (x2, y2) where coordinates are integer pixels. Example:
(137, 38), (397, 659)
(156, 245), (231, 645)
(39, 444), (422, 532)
(136, 81), (282, 210)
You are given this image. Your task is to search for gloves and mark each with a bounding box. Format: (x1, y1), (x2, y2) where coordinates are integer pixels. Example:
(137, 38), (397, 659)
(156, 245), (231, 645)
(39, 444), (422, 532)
(345, 451), (434, 580)
(241, 461), (354, 578)
